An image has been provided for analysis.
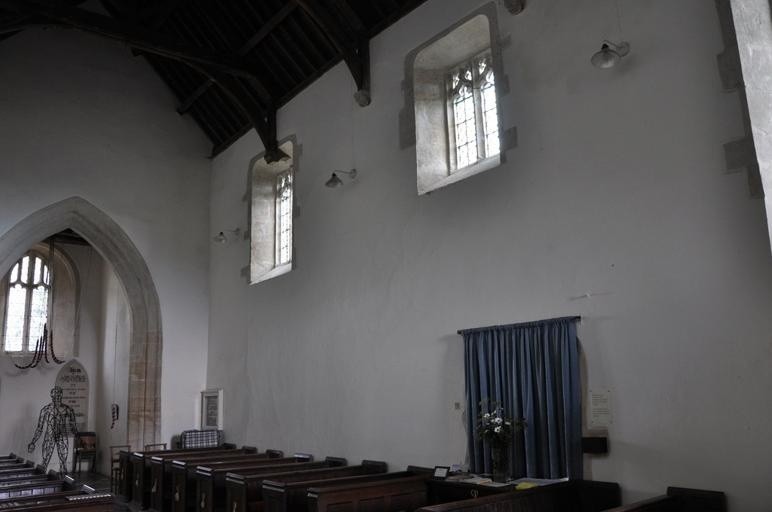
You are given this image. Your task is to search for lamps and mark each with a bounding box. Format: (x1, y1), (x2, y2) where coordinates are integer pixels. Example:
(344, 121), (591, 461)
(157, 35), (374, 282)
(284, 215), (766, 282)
(212, 228), (240, 245)
(590, 38), (630, 69)
(13, 235), (64, 370)
(325, 170), (356, 188)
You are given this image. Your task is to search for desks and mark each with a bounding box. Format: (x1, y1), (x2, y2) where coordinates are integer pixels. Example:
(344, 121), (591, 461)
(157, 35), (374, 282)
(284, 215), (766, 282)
(0, 453), (115, 512)
(119, 442), (730, 512)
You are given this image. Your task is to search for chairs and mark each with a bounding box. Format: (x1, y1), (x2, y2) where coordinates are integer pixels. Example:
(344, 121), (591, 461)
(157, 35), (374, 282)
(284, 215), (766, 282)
(109, 445), (133, 492)
(72, 431), (97, 477)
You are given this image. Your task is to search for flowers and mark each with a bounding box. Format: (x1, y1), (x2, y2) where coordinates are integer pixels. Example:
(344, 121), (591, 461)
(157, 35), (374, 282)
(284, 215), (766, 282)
(475, 399), (530, 458)
